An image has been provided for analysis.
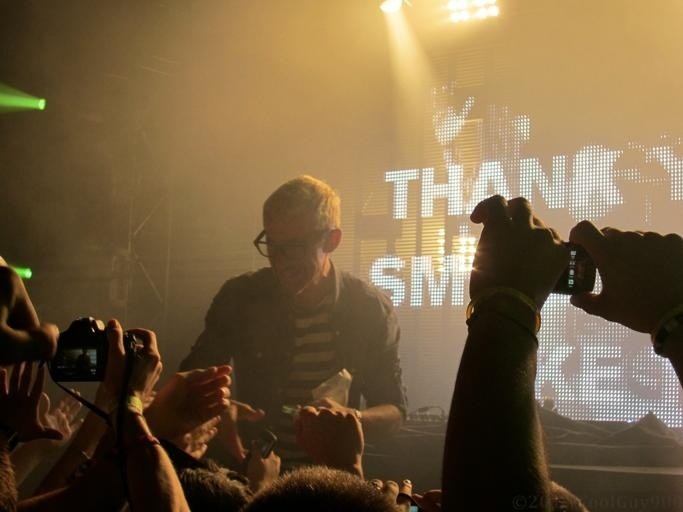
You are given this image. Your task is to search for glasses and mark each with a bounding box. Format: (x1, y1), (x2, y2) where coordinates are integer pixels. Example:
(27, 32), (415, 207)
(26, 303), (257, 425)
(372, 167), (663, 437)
(253, 230), (328, 260)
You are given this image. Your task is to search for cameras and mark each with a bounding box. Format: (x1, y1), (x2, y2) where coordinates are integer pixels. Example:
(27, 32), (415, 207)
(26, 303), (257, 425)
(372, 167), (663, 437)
(51, 316), (137, 381)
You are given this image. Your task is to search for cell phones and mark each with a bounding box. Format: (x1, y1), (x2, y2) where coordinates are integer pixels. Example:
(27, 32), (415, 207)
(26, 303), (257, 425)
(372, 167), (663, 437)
(552, 242), (596, 294)
(256, 428), (278, 458)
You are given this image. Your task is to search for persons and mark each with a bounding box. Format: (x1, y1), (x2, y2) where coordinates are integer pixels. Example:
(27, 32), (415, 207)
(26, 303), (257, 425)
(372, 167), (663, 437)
(1, 178), (683, 512)
